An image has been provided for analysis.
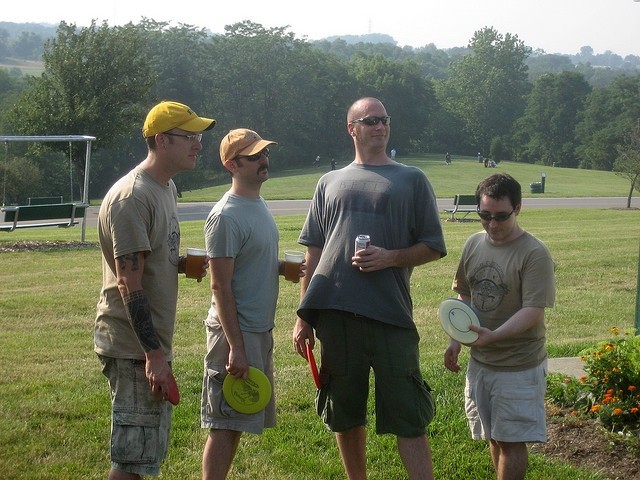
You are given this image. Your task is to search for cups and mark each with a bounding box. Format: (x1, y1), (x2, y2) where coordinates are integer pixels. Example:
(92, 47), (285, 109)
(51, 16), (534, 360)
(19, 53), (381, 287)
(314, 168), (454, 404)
(186, 248), (207, 278)
(284, 250), (306, 281)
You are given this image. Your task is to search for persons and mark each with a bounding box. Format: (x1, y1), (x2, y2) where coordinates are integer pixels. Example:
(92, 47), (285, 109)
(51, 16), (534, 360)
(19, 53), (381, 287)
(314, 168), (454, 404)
(439, 174), (557, 480)
(329, 157), (337, 170)
(483, 157), (496, 168)
(445, 152), (451, 165)
(390, 147), (397, 160)
(200, 128), (308, 479)
(290, 97), (447, 480)
(90, 99), (216, 480)
(313, 155), (321, 167)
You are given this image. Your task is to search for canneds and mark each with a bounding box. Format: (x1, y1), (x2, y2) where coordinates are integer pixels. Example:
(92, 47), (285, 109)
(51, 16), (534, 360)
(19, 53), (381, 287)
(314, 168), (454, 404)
(354, 234), (371, 256)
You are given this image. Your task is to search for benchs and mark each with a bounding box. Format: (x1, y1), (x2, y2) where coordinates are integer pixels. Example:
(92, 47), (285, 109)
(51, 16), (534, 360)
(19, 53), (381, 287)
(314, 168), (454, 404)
(444, 194), (479, 222)
(0, 195), (87, 232)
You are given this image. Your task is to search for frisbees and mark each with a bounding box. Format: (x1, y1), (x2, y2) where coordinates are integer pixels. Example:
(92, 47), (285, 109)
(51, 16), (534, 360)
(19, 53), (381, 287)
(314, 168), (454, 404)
(168, 374), (180, 405)
(439, 298), (480, 344)
(223, 366), (272, 414)
(306, 343), (322, 389)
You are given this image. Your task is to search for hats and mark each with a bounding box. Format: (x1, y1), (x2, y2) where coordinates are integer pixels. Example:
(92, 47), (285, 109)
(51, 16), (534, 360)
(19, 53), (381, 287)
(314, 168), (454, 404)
(220, 128), (278, 165)
(142, 101), (216, 138)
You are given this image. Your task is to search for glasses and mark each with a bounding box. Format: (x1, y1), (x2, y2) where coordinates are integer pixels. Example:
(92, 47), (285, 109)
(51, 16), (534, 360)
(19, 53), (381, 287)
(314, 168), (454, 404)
(231, 148), (270, 162)
(348, 116), (391, 126)
(162, 132), (202, 144)
(477, 204), (516, 222)
